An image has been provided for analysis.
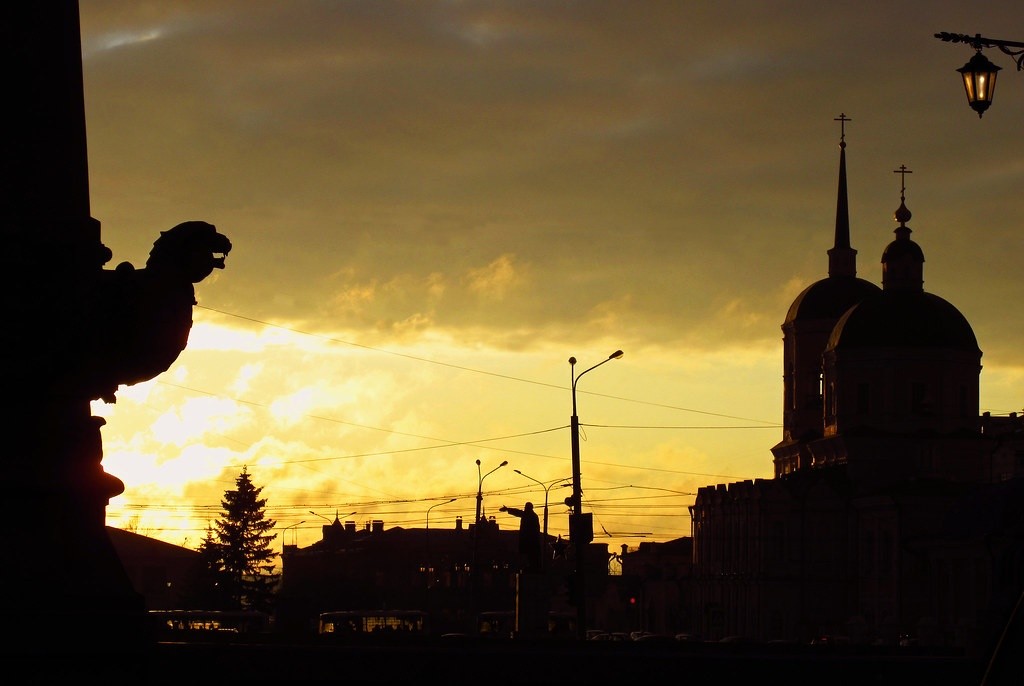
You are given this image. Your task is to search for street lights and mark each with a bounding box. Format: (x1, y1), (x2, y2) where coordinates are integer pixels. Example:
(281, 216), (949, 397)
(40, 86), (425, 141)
(516, 470), (574, 564)
(565, 349), (624, 639)
(470, 458), (511, 578)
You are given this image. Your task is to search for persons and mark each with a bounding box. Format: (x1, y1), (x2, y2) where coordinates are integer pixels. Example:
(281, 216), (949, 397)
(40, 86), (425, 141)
(501, 502), (541, 570)
